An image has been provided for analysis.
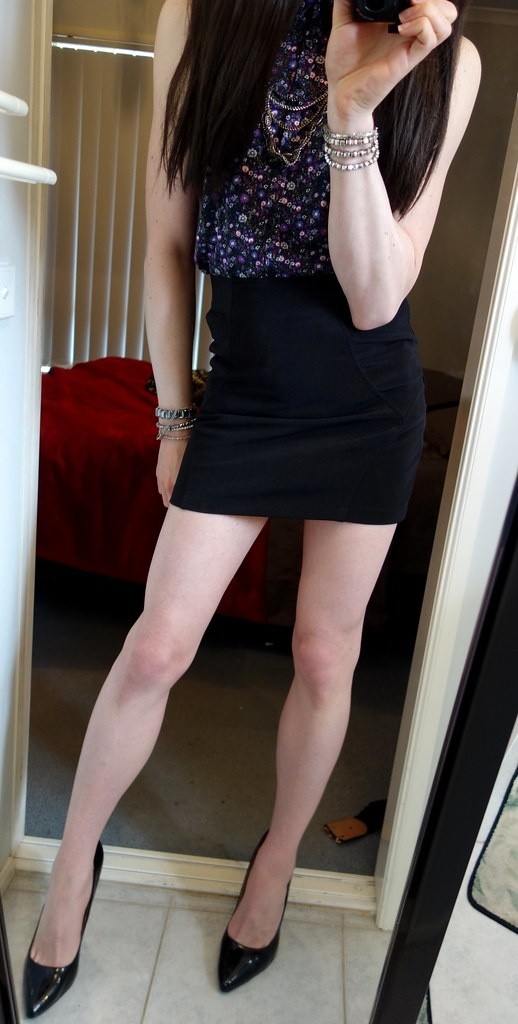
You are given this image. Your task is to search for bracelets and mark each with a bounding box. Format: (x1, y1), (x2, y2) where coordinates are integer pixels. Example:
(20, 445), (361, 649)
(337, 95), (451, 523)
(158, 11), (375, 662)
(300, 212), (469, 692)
(323, 126), (377, 145)
(155, 404), (198, 419)
(156, 421), (194, 440)
(325, 146), (379, 171)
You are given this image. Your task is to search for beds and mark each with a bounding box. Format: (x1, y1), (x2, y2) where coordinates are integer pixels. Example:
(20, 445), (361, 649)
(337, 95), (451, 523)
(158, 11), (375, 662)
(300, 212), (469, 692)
(37, 339), (465, 706)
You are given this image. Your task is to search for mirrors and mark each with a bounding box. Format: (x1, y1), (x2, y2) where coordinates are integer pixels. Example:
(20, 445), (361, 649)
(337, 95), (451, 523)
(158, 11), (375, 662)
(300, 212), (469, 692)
(0, 1), (518, 1023)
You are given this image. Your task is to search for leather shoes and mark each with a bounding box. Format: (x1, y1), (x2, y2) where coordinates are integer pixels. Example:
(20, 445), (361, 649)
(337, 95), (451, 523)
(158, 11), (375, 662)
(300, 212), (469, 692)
(219, 825), (293, 993)
(22, 839), (105, 1018)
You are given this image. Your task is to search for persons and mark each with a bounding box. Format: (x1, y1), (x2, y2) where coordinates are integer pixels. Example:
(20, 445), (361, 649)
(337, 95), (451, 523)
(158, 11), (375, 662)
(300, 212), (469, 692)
(23, 0), (480, 1020)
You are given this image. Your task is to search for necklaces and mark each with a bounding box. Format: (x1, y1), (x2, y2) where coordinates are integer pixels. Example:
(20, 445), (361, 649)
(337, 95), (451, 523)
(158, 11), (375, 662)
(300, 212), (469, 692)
(266, 91), (328, 167)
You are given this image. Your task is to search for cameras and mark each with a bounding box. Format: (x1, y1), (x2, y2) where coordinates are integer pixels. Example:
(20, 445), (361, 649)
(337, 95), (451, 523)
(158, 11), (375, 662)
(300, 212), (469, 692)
(352, 0), (412, 24)
